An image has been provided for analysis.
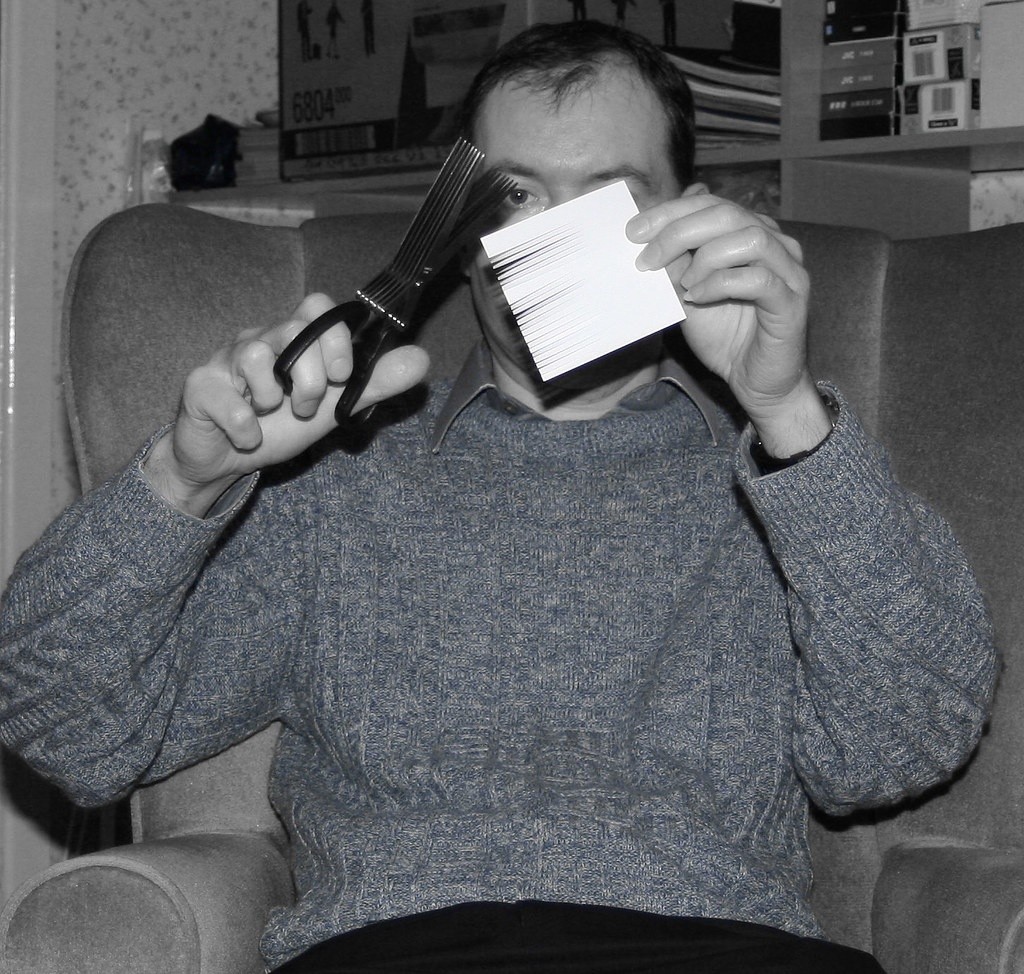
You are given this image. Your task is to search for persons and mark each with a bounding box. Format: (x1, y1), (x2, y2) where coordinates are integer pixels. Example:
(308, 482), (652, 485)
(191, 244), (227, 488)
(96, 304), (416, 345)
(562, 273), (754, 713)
(0, 19), (999, 974)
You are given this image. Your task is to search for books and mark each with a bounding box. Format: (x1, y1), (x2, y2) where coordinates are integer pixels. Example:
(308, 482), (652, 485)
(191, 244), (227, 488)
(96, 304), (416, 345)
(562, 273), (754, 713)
(657, 47), (782, 143)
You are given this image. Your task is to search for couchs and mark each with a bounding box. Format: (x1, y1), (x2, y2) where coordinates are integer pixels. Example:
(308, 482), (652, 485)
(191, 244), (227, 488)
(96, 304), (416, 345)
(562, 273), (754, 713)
(0, 194), (1024, 973)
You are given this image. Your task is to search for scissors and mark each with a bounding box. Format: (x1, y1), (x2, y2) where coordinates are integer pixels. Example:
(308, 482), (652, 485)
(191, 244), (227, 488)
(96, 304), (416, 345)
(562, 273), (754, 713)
(271, 134), (520, 430)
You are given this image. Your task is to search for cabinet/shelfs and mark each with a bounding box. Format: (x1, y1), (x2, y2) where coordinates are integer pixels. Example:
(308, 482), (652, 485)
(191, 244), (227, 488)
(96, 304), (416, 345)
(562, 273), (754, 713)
(276, 0), (1024, 184)
